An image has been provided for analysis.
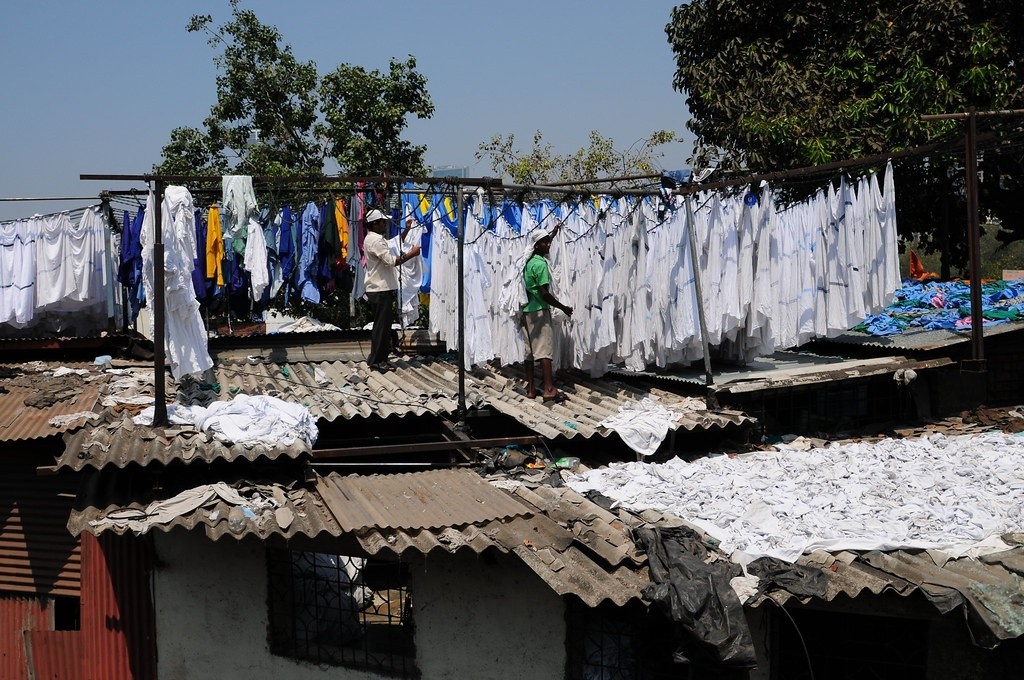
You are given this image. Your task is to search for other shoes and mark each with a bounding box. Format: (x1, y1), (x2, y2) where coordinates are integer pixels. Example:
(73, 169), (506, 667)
(369, 363), (396, 372)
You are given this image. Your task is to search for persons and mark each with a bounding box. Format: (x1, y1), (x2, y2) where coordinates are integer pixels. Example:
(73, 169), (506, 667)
(360, 210), (423, 372)
(517, 228), (573, 402)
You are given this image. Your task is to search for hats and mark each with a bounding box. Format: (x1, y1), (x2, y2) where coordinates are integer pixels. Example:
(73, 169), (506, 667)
(532, 228), (549, 242)
(366, 210), (392, 222)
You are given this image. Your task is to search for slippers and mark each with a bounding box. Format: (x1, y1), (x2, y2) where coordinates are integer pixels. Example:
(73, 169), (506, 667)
(543, 389), (569, 401)
(527, 390), (540, 399)
